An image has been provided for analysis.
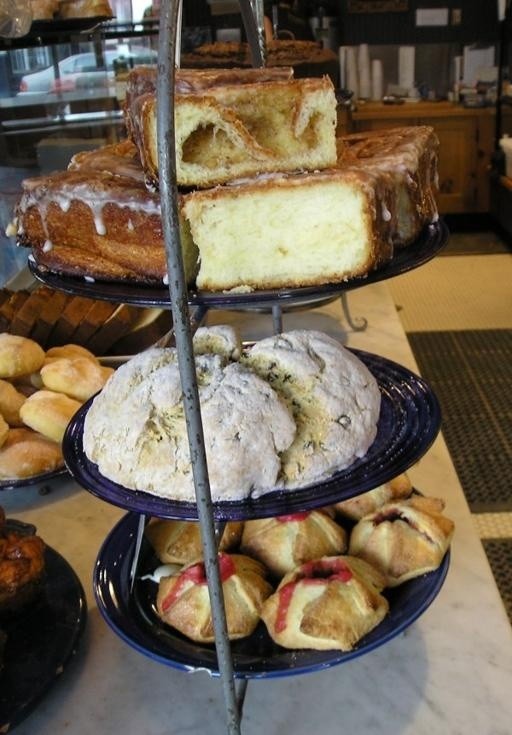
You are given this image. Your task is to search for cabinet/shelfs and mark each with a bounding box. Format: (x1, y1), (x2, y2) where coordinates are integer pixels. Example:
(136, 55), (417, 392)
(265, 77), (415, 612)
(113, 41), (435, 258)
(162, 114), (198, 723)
(25, 1), (458, 735)
(1, 2), (240, 162)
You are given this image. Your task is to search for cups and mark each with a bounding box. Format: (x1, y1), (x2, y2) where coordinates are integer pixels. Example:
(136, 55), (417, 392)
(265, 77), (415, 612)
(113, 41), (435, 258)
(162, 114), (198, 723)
(335, 41), (417, 102)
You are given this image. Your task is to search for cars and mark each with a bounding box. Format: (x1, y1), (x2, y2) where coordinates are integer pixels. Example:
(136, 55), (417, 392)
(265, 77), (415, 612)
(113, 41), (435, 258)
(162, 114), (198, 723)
(15, 48), (160, 101)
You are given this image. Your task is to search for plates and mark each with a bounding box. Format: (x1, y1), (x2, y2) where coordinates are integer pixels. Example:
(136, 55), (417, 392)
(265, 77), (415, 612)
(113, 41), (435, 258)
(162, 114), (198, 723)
(95, 510), (451, 684)
(1, 453), (73, 490)
(177, 51), (336, 83)
(25, 220), (450, 319)
(2, 519), (88, 730)
(62, 348), (444, 523)
(1, 268), (202, 364)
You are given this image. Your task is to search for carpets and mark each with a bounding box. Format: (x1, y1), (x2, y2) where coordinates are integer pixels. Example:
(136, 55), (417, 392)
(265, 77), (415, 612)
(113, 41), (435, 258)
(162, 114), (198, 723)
(407, 328), (512, 512)
(481, 537), (512, 633)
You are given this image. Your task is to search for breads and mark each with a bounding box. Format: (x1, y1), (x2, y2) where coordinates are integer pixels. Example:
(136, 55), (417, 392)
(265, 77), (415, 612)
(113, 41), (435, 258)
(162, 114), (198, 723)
(0, 0), (453, 653)
